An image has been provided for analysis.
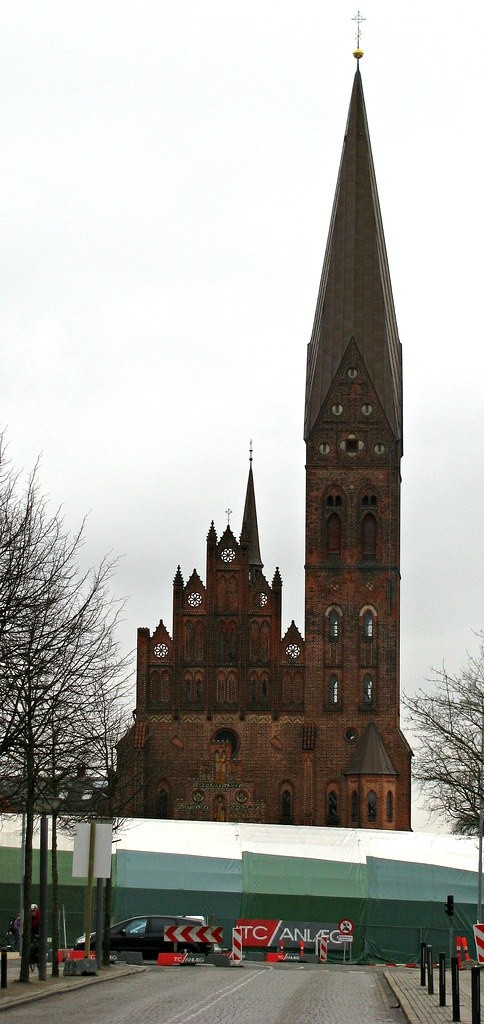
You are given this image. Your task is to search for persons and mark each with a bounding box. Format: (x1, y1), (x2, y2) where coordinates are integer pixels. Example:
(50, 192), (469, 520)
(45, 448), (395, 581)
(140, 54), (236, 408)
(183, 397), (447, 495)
(30, 904), (40, 968)
(11, 913), (20, 952)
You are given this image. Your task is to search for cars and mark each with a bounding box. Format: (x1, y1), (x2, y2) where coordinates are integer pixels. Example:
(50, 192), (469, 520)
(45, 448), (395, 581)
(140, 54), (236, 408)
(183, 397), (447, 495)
(73, 915), (213, 965)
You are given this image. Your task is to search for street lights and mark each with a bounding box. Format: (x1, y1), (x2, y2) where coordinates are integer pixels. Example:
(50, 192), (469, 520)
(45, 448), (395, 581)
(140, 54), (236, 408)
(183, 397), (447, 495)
(87, 816), (116, 971)
(35, 796), (61, 981)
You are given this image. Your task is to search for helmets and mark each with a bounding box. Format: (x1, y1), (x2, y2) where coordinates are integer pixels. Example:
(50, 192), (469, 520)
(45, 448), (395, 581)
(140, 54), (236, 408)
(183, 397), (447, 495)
(31, 903), (38, 911)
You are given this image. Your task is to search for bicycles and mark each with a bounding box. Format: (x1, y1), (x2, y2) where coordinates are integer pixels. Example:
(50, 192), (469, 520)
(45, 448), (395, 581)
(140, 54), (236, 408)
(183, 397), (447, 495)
(10, 929), (50, 972)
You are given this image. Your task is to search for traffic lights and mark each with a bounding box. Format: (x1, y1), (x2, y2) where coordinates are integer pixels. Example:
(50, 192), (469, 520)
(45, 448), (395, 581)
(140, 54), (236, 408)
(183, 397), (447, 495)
(444, 895), (454, 916)
